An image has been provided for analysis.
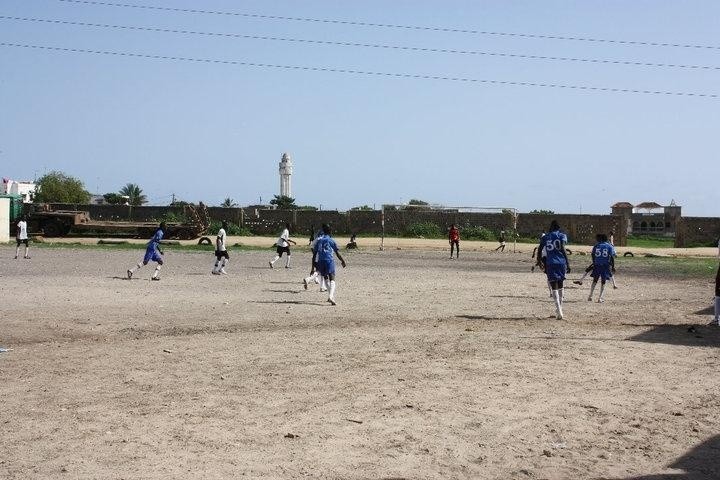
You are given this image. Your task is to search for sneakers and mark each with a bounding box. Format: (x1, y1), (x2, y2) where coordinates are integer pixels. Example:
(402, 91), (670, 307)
(556, 307), (563, 319)
(573, 280), (582, 285)
(212, 269), (227, 275)
(587, 295), (593, 301)
(152, 276), (161, 280)
(598, 298), (604, 303)
(706, 319), (720, 325)
(613, 285), (618, 289)
(303, 278), (327, 293)
(327, 297), (336, 305)
(285, 265), (291, 268)
(127, 269), (132, 279)
(24, 256), (31, 259)
(15, 256), (17, 259)
(269, 261), (273, 268)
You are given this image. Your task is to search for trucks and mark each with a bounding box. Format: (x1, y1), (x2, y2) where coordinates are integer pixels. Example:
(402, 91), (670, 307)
(0, 193), (213, 242)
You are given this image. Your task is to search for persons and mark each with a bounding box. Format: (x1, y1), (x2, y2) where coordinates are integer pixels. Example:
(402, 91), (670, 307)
(530, 219), (618, 320)
(448, 224), (460, 259)
(496, 230), (506, 252)
(708, 261), (720, 326)
(14, 214), (32, 259)
(127, 222), (168, 281)
(212, 221), (230, 275)
(303, 222), (346, 306)
(269, 223), (296, 269)
